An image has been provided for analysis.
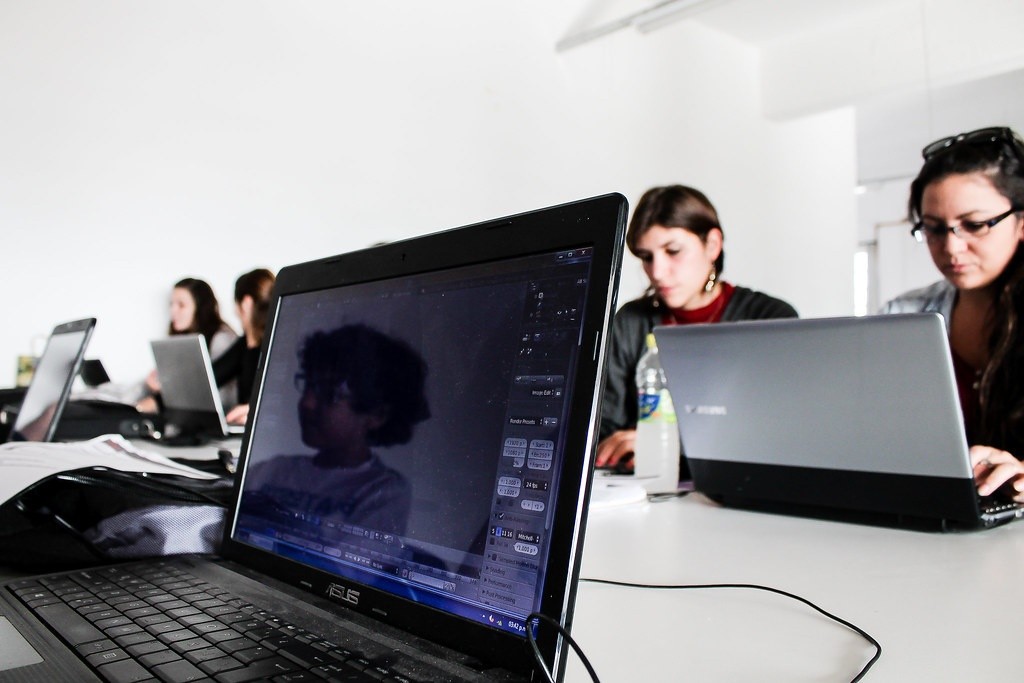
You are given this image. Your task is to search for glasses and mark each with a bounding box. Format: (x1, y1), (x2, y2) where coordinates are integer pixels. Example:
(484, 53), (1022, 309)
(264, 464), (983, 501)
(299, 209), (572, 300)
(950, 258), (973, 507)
(922, 127), (1012, 166)
(911, 206), (1024, 244)
(293, 373), (350, 404)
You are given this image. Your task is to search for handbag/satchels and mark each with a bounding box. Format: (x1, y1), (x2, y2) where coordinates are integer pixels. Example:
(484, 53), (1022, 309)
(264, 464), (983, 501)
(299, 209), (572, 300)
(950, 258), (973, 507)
(0, 464), (237, 568)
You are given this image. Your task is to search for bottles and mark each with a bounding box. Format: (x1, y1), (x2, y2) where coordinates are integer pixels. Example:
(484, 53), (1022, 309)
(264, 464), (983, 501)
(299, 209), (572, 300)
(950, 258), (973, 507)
(634, 333), (680, 495)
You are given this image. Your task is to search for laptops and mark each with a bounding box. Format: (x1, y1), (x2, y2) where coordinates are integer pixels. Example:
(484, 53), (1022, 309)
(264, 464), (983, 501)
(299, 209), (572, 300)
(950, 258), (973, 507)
(150, 334), (245, 439)
(652, 312), (1024, 534)
(0, 317), (95, 445)
(0, 192), (628, 682)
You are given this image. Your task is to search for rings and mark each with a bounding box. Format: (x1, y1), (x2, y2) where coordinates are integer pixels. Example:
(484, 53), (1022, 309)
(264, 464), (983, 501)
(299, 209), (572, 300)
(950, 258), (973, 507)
(979, 460), (993, 471)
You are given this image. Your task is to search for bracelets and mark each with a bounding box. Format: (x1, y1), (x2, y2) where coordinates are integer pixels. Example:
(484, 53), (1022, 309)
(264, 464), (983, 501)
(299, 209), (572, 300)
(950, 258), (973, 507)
(136, 405), (143, 412)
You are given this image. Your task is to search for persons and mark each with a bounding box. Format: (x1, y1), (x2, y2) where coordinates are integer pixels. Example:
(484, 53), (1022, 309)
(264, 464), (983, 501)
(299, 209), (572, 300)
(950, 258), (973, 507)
(594, 186), (799, 470)
(136, 269), (276, 425)
(246, 322), (433, 537)
(878, 127), (1024, 502)
